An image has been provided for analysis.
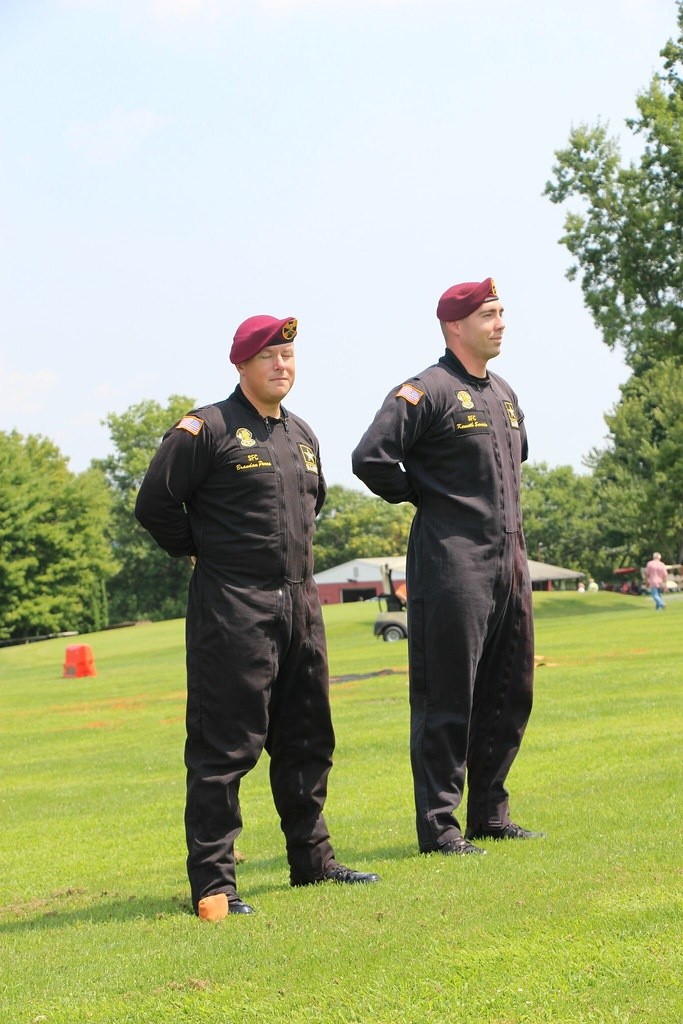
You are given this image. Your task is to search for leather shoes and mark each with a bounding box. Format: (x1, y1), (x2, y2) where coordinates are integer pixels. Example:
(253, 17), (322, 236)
(325, 865), (380, 884)
(420, 837), (485, 854)
(464, 823), (546, 840)
(225, 892), (253, 914)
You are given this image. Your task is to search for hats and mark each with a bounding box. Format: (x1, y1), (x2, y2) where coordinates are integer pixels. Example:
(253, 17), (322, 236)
(230, 315), (297, 364)
(436, 278), (499, 321)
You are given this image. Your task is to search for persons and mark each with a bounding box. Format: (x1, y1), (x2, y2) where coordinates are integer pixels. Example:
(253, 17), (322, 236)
(352, 277), (538, 854)
(575, 577), (598, 592)
(133, 314), (379, 918)
(645, 552), (668, 609)
